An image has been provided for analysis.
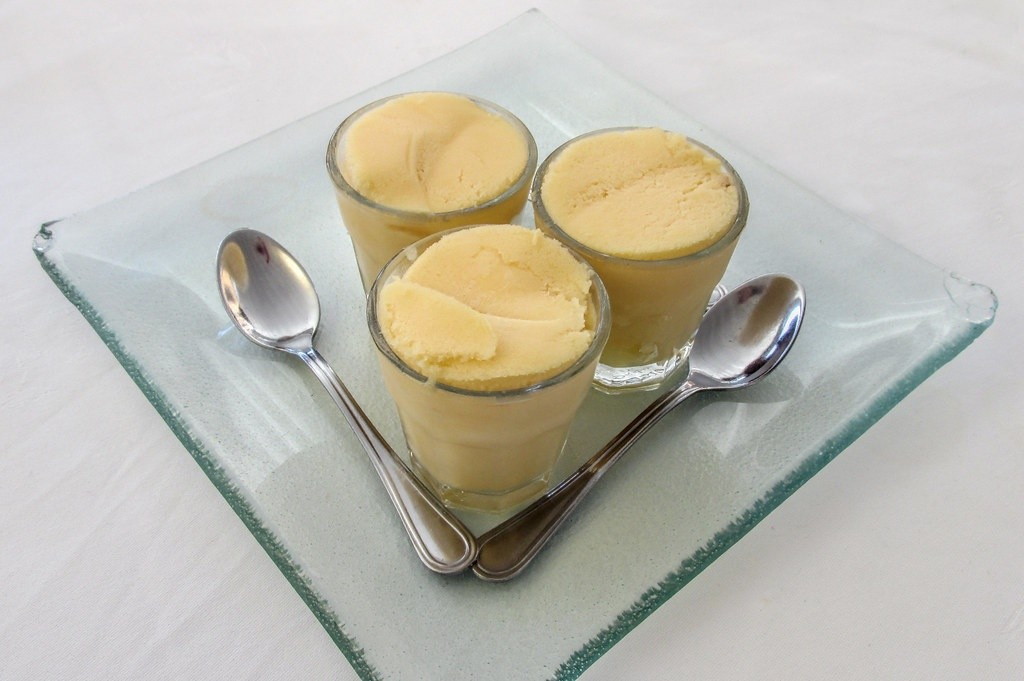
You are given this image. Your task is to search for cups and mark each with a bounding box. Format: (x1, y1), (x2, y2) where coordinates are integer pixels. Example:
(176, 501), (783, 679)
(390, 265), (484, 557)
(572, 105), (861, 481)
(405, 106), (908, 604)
(529, 125), (751, 394)
(324, 89), (540, 299)
(366, 223), (612, 516)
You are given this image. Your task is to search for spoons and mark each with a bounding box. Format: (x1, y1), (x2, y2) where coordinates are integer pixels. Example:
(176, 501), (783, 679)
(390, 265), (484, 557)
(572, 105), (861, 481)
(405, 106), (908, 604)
(470, 273), (807, 583)
(215, 228), (481, 575)
